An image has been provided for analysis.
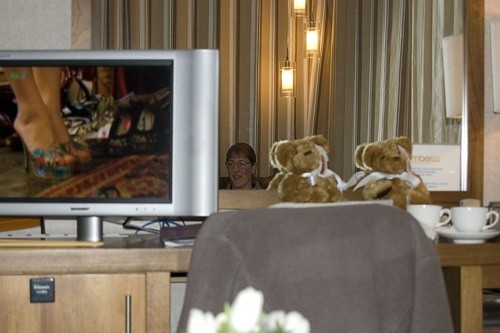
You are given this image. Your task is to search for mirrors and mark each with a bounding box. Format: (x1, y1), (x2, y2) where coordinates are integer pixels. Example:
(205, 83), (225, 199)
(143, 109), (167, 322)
(0, 0), (484, 205)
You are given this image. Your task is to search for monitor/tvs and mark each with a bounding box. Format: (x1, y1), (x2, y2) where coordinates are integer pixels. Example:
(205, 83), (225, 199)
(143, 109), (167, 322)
(0, 47), (219, 218)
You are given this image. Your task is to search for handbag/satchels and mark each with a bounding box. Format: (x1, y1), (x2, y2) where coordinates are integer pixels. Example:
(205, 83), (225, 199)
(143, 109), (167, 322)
(65, 86), (172, 155)
(60, 76), (100, 120)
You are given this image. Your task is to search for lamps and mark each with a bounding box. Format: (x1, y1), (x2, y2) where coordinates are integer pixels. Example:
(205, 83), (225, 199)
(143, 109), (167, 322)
(281, 58), (294, 94)
(293, 0), (307, 18)
(303, 21), (321, 58)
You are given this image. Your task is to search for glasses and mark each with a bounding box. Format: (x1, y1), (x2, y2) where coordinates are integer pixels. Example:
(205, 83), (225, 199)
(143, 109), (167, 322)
(225, 160), (252, 169)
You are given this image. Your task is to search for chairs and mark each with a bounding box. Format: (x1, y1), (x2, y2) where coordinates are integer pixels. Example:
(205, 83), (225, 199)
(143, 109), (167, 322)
(172, 206), (454, 333)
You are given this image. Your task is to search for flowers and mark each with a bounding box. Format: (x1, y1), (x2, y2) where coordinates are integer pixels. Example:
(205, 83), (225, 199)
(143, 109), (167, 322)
(186, 285), (310, 333)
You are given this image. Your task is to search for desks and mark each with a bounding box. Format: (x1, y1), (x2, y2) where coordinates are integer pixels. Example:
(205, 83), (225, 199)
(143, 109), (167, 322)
(0, 193), (500, 333)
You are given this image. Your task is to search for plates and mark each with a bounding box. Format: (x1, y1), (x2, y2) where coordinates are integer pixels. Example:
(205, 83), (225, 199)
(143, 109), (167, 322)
(436, 227), (500, 244)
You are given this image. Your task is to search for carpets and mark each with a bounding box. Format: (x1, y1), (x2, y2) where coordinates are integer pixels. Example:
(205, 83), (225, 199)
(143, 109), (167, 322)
(33, 155), (170, 199)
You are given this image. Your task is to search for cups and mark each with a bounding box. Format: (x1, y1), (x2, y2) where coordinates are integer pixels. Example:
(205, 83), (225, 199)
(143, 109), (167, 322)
(450, 207), (500, 232)
(407, 205), (450, 240)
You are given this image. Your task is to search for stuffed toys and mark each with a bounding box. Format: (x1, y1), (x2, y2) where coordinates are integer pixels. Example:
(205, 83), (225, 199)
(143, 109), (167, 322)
(276, 135), (341, 203)
(344, 142), (372, 191)
(362, 137), (432, 211)
(267, 139), (291, 191)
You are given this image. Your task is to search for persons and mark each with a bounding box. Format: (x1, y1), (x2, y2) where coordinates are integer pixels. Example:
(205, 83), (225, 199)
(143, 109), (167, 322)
(1, 67), (92, 176)
(223, 143), (265, 190)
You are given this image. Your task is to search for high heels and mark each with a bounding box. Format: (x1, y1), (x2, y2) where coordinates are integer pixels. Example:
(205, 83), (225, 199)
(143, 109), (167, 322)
(13, 124), (91, 180)
